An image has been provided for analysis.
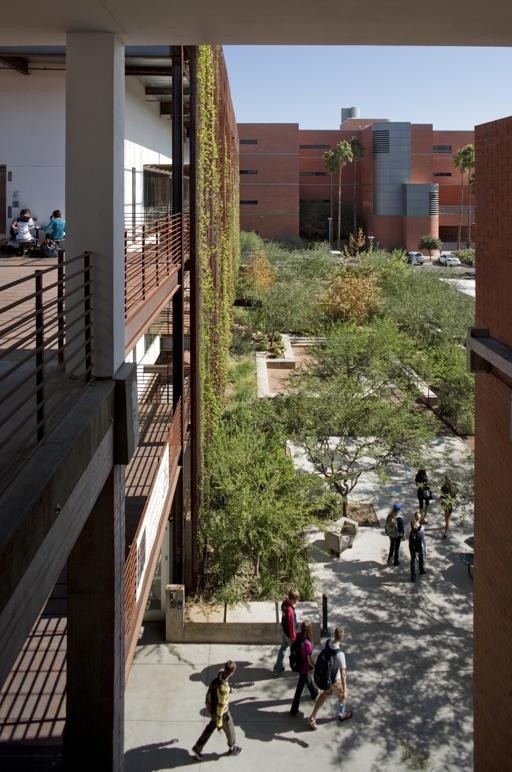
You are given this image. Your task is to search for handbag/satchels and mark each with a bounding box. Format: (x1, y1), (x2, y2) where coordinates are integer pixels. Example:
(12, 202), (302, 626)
(41, 237), (59, 258)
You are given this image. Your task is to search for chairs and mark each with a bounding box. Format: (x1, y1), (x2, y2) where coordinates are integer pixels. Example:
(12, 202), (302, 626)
(326, 517), (358, 557)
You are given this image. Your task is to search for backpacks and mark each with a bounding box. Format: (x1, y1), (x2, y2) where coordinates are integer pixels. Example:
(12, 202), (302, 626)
(205, 677), (222, 717)
(313, 635), (345, 692)
(408, 520), (424, 554)
(288, 634), (307, 676)
(385, 513), (403, 539)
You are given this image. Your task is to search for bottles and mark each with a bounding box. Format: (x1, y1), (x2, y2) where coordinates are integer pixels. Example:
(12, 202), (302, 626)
(337, 702), (345, 717)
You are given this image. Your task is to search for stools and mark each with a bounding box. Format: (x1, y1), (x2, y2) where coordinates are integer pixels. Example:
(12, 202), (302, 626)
(17, 239), (29, 256)
(53, 240), (64, 248)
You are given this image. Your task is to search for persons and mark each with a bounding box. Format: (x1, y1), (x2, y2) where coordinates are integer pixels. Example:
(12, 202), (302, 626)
(274, 588), (300, 671)
(15, 209), (37, 255)
(407, 512), (427, 581)
(192, 660), (242, 759)
(385, 503), (405, 565)
(307, 627), (354, 729)
(440, 482), (453, 537)
(289, 621), (320, 717)
(44, 215), (65, 239)
(415, 467), (432, 524)
(10, 212), (24, 239)
(42, 210), (65, 250)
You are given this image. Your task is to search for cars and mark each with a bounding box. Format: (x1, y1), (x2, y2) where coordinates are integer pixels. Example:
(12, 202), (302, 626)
(438, 253), (460, 268)
(406, 251), (425, 266)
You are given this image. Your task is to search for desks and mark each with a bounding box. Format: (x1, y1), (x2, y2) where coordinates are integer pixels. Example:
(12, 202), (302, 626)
(29, 226), (52, 247)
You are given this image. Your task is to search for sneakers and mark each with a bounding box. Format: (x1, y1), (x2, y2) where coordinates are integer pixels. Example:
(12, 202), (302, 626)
(288, 705), (352, 732)
(385, 557), (401, 567)
(190, 746), (242, 762)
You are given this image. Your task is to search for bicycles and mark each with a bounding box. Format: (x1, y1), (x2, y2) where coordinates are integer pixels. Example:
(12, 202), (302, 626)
(459, 553), (473, 579)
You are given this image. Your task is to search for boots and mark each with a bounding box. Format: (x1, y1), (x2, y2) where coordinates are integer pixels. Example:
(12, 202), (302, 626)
(419, 504), (429, 524)
